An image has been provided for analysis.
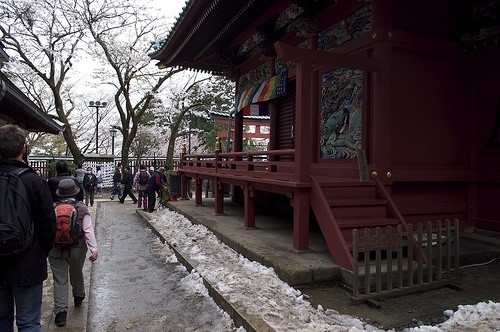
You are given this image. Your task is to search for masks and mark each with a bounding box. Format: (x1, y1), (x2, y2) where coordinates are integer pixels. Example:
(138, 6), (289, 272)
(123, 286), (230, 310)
(87, 170), (92, 173)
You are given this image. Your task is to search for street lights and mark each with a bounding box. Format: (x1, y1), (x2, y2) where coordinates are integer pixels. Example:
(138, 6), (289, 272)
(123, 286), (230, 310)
(110, 126), (117, 157)
(88, 100), (107, 153)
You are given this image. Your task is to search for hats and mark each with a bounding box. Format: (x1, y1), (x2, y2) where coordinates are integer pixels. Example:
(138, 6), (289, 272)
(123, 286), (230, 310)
(56, 179), (80, 197)
(157, 167), (165, 173)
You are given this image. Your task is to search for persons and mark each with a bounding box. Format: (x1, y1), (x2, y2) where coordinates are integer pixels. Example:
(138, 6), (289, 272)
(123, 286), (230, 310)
(110, 163), (123, 200)
(48, 161), (84, 204)
(120, 166), (138, 204)
(83, 167), (98, 207)
(95, 166), (103, 197)
(0, 123), (57, 332)
(151, 163), (164, 198)
(134, 164), (151, 210)
(47, 178), (98, 327)
(145, 166), (164, 213)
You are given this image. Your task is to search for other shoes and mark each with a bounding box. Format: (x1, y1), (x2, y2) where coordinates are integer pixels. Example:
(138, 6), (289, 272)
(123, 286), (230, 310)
(54, 310), (67, 326)
(120, 198), (124, 203)
(74, 297), (84, 307)
(110, 195), (115, 200)
(132, 199), (138, 204)
(118, 196), (121, 199)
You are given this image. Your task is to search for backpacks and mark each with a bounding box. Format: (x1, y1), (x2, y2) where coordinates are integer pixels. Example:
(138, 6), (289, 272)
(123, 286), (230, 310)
(53, 201), (82, 247)
(0, 168), (34, 258)
(138, 171), (148, 184)
